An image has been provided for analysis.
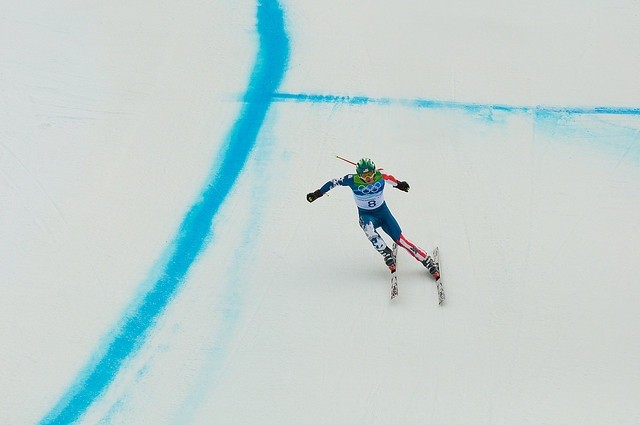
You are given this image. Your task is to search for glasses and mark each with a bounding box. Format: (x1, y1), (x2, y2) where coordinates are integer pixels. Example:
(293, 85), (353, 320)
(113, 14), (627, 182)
(357, 170), (376, 181)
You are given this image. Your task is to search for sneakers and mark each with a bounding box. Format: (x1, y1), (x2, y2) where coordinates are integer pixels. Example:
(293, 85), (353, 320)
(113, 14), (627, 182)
(421, 255), (438, 275)
(380, 245), (395, 267)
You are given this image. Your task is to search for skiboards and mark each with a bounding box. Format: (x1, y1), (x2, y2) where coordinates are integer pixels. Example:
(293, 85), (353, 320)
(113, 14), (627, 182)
(389, 243), (446, 306)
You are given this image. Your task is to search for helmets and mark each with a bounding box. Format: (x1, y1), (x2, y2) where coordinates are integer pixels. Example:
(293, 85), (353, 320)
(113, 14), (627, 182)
(355, 156), (377, 184)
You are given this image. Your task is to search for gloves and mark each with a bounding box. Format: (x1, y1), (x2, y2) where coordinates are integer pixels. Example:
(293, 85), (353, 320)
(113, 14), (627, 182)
(392, 179), (411, 192)
(306, 189), (324, 203)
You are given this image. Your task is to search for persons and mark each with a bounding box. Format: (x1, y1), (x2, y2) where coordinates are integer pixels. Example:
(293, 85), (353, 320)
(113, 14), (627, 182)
(305, 158), (439, 280)
